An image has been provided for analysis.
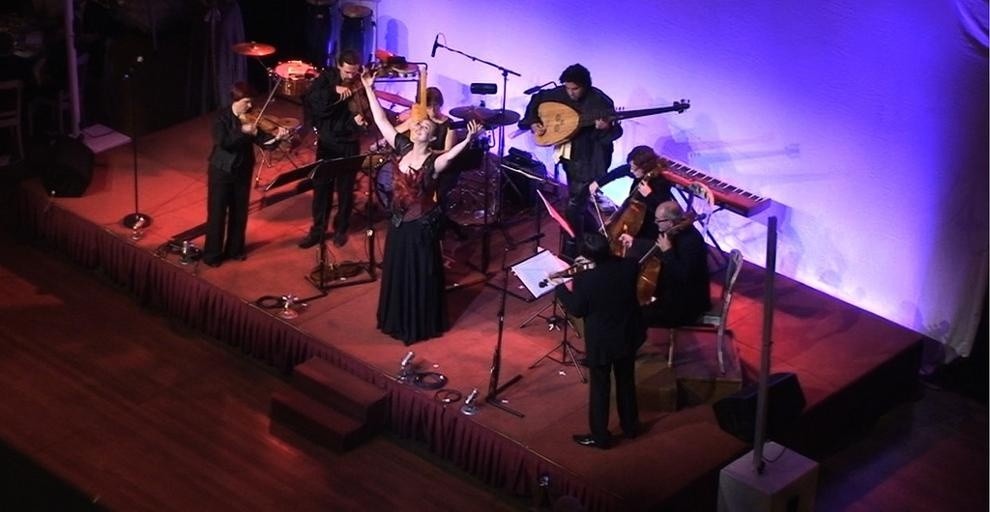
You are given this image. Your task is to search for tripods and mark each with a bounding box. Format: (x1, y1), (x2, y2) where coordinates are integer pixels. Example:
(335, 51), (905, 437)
(528, 280), (588, 384)
(517, 297), (582, 341)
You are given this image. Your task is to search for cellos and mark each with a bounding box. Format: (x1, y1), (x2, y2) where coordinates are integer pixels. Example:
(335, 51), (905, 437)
(604, 158), (670, 256)
(634, 210), (696, 303)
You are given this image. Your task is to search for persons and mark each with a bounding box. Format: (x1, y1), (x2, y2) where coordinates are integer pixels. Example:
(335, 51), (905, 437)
(360, 65), (483, 346)
(369, 88), (468, 195)
(618, 200), (712, 330)
(518, 63), (623, 216)
(0, 0), (122, 168)
(204, 81), (289, 267)
(588, 146), (670, 263)
(547, 232), (647, 450)
(299, 50), (369, 249)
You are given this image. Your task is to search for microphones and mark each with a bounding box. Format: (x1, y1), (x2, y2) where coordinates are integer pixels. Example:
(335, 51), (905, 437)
(523, 81), (554, 95)
(517, 232), (546, 245)
(430, 31), (441, 58)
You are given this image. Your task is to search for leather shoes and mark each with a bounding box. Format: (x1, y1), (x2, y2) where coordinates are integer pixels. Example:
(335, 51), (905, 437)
(573, 434), (598, 446)
(333, 230), (345, 247)
(207, 250), (246, 268)
(298, 236), (319, 248)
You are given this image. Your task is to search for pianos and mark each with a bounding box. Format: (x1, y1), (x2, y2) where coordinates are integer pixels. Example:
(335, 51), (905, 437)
(656, 151), (770, 217)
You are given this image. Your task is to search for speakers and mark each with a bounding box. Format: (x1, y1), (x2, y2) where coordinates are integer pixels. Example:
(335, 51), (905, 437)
(41, 140), (96, 198)
(714, 370), (808, 440)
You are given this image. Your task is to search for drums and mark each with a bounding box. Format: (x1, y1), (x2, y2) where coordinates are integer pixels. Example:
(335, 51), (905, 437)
(374, 154), (396, 212)
(445, 162), (500, 226)
(272, 60), (320, 98)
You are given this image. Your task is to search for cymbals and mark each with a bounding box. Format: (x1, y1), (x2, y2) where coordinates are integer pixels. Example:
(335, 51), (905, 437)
(373, 89), (413, 107)
(449, 105), (498, 121)
(488, 108), (520, 125)
(230, 42), (277, 57)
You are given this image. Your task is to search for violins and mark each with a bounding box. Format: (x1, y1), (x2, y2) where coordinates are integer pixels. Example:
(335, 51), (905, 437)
(239, 104), (299, 140)
(540, 258), (600, 291)
(340, 74), (373, 133)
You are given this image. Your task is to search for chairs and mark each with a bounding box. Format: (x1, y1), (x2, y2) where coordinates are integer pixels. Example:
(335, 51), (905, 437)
(665, 247), (745, 377)
(0, 80), (27, 161)
